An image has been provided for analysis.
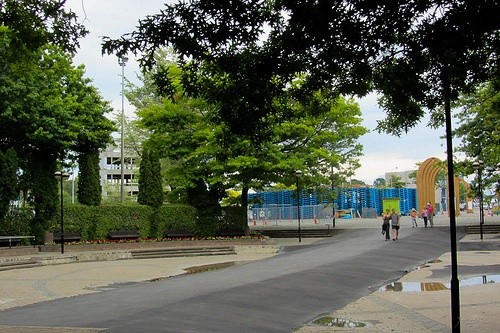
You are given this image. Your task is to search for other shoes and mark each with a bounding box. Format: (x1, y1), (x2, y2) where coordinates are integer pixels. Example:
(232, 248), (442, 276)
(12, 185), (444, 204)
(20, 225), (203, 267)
(392, 238), (396, 241)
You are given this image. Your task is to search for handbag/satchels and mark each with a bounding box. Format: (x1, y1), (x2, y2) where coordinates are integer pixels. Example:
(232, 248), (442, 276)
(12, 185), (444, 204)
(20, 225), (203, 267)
(382, 223), (386, 230)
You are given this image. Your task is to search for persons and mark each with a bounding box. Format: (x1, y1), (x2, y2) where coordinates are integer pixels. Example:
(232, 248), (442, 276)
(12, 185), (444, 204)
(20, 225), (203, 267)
(410, 208), (419, 228)
(488, 210), (494, 219)
(382, 208), (401, 241)
(259, 209), (272, 226)
(421, 201), (435, 228)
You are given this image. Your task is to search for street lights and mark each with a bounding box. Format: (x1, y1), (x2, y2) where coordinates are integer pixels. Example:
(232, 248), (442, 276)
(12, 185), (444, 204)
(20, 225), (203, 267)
(472, 160), (484, 239)
(390, 167), (398, 187)
(291, 169), (302, 242)
(68, 171), (74, 203)
(54, 171), (70, 254)
(118, 58), (128, 203)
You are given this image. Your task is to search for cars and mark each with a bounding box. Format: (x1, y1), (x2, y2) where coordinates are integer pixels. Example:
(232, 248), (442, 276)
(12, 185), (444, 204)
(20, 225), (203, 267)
(460, 199), (497, 211)
